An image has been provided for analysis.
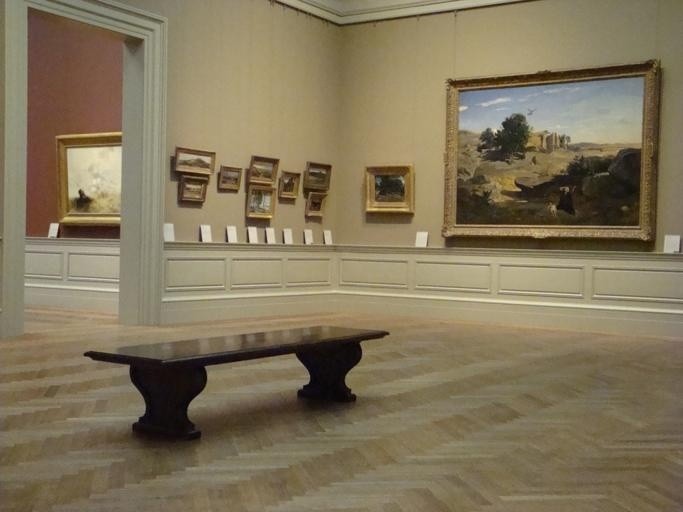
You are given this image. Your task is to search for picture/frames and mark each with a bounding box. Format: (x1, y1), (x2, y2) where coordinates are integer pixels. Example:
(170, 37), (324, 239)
(439, 58), (657, 241)
(365, 165), (416, 216)
(172, 142), (335, 223)
(51, 129), (123, 228)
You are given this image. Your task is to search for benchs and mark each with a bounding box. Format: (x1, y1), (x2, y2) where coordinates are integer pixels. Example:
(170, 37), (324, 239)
(84, 324), (393, 442)
